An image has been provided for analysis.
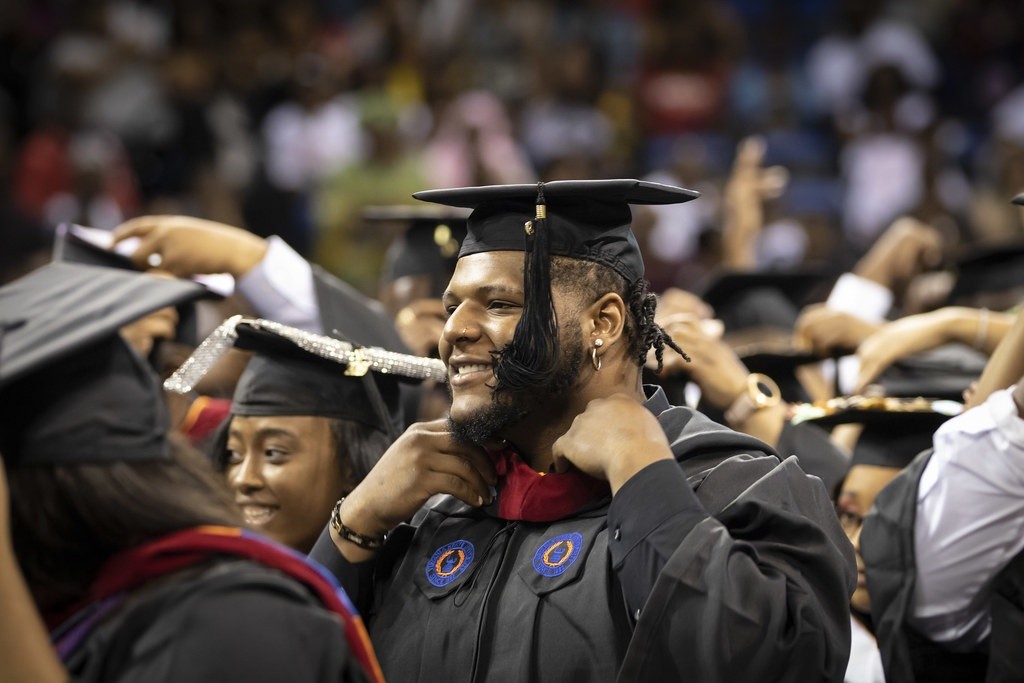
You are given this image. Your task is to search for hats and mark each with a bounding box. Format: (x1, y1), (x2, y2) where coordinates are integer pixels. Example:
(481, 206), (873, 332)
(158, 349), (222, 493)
(790, 396), (961, 470)
(0, 263), (208, 465)
(705, 269), (824, 333)
(412, 179), (700, 285)
(362, 205), (470, 296)
(921, 243), (1022, 313)
(696, 342), (859, 421)
(56, 219), (232, 349)
(229, 319), (443, 442)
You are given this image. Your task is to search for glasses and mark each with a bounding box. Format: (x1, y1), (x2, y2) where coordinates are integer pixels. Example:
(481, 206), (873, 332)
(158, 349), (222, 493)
(835, 503), (866, 527)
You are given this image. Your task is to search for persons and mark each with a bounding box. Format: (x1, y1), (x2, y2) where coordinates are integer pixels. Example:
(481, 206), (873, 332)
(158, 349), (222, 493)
(859, 376), (1024, 681)
(0, 257), (382, 680)
(221, 315), (403, 563)
(4, 20), (1024, 460)
(661, 309), (972, 683)
(298, 176), (858, 683)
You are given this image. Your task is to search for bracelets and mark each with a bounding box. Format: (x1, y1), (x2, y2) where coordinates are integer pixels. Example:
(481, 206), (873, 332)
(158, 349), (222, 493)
(330, 497), (386, 550)
